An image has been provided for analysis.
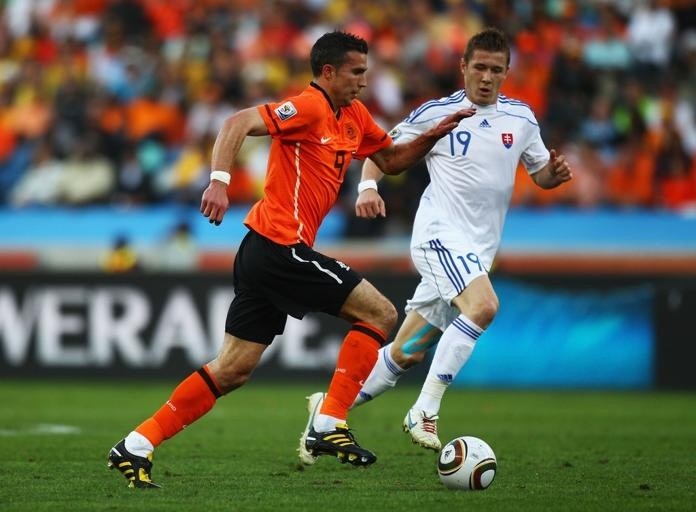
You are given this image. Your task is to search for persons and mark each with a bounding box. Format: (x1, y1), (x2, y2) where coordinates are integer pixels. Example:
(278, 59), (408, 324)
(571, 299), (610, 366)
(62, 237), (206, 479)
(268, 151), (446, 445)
(296, 28), (574, 467)
(0, 0), (696, 207)
(104, 26), (475, 487)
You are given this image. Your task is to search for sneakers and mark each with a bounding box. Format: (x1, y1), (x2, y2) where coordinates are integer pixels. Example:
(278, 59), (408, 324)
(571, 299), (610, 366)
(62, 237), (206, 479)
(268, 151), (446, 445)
(401, 408), (442, 452)
(105, 437), (162, 491)
(305, 426), (376, 468)
(297, 390), (332, 464)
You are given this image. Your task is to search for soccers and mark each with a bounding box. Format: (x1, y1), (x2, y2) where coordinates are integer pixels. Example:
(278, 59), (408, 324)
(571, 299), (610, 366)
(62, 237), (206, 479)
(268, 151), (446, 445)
(437, 435), (497, 489)
